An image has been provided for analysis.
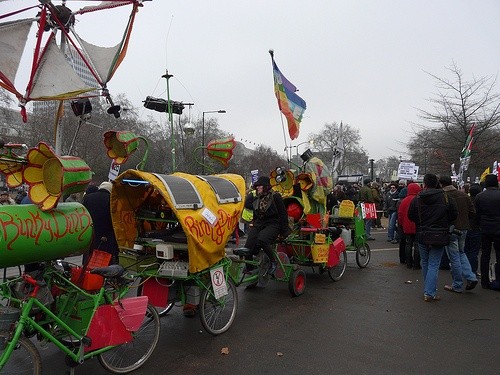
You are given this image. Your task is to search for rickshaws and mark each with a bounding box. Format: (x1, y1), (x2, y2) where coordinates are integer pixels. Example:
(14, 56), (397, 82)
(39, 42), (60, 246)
(229, 198), (372, 297)
(0, 168), (251, 375)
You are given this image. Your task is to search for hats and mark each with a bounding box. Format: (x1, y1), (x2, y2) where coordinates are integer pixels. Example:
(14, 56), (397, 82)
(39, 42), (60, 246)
(253, 176), (270, 186)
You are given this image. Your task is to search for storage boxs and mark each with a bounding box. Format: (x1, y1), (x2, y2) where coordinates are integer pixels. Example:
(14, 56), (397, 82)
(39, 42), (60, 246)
(71, 248), (111, 289)
(55, 293), (106, 335)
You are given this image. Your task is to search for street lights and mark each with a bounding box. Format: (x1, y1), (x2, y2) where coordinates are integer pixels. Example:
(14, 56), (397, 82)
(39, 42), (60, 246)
(202, 110), (226, 175)
(297, 140), (313, 172)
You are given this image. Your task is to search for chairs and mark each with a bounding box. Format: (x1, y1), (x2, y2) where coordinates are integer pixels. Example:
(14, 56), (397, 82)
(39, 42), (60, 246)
(135, 215), (188, 251)
(329, 205), (362, 219)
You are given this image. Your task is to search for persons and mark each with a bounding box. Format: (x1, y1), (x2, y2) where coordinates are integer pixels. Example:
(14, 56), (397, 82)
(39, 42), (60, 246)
(0, 184), (41, 274)
(326, 178), (409, 244)
(398, 174), (500, 302)
(231, 176), (289, 290)
(66, 180), (120, 268)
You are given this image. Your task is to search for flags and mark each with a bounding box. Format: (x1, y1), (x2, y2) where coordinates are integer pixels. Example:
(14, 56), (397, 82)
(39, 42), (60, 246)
(271, 58), (306, 141)
(464, 137), (474, 156)
(479, 167), (490, 183)
(462, 124), (475, 152)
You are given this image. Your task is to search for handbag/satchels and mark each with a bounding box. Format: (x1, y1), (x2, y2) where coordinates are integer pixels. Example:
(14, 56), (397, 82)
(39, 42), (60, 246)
(420, 224), (451, 250)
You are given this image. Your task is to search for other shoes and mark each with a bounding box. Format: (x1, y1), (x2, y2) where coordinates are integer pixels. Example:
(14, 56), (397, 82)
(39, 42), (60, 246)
(444, 284), (462, 294)
(423, 293), (441, 303)
(476, 270), (500, 291)
(465, 279), (478, 290)
(268, 262), (277, 274)
